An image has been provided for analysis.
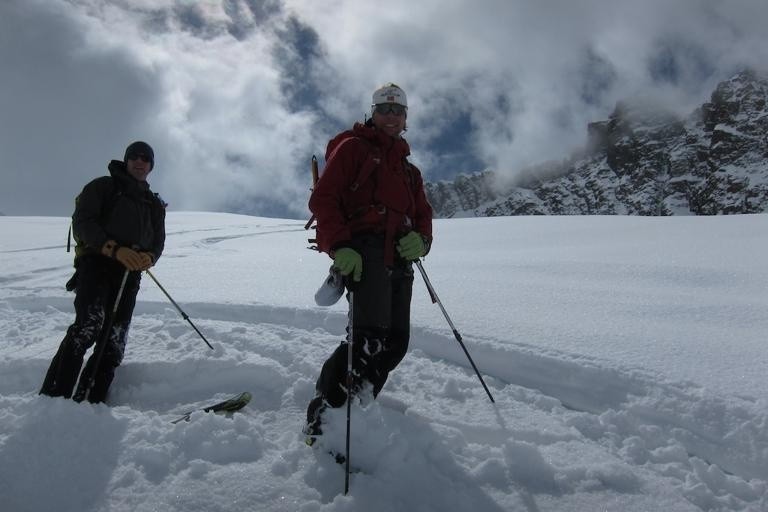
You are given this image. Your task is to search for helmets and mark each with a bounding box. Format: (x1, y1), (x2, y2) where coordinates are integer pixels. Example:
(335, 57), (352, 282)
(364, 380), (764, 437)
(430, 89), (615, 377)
(128, 154), (149, 161)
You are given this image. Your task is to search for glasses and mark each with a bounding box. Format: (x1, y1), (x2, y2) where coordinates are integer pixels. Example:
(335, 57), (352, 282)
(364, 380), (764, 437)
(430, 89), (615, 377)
(376, 104), (405, 116)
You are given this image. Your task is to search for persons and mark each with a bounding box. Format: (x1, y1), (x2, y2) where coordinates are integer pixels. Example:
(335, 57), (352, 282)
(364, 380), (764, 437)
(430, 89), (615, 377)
(38, 141), (164, 403)
(302, 82), (433, 446)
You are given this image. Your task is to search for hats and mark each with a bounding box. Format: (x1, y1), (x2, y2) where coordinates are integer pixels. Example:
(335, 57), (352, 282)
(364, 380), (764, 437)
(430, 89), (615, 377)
(371, 86), (407, 109)
(124, 141), (154, 172)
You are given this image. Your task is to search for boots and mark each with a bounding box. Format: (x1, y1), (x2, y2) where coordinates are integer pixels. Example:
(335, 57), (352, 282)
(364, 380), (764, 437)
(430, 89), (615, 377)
(39, 345), (120, 404)
(302, 340), (392, 474)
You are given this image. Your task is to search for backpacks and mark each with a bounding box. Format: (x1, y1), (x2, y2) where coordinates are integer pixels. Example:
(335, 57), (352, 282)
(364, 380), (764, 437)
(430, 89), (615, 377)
(72, 195), (95, 268)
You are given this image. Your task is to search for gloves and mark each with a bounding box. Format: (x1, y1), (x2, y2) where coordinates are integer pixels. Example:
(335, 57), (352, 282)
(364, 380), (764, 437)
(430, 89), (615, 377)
(102, 240), (155, 271)
(332, 248), (362, 282)
(396, 231), (427, 261)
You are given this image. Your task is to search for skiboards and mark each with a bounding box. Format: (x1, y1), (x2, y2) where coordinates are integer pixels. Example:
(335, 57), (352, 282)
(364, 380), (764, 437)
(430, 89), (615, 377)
(171, 391), (251, 424)
(304, 437), (362, 475)
